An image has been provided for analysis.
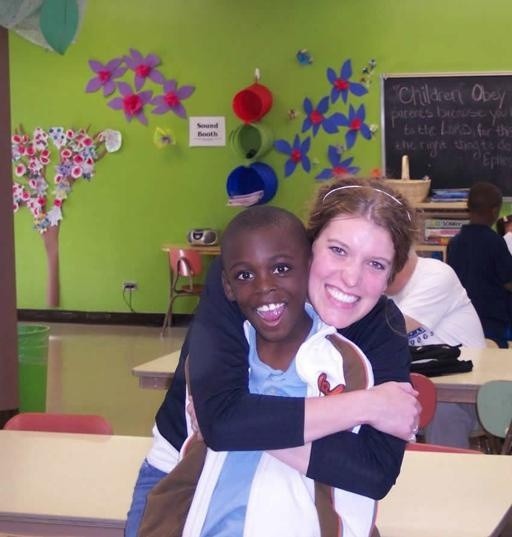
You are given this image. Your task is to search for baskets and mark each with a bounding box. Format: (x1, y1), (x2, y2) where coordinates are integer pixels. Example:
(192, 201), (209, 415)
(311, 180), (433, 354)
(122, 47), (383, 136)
(383, 155), (431, 204)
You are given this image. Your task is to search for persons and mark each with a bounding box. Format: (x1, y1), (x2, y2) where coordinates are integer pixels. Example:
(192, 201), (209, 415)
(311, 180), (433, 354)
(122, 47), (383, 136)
(444, 180), (511, 348)
(134, 205), (378, 537)
(382, 244), (485, 450)
(495, 215), (511, 254)
(119, 174), (423, 536)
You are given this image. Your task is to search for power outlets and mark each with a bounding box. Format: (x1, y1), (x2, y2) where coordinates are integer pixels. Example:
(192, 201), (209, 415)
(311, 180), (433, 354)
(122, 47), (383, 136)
(122, 281), (137, 290)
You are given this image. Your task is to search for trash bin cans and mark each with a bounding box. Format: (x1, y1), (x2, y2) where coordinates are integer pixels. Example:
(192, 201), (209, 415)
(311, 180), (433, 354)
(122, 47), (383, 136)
(18, 324), (50, 412)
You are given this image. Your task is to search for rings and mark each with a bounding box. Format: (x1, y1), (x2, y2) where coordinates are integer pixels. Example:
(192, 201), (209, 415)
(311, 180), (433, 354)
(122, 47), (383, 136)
(407, 434), (417, 441)
(192, 420), (198, 432)
(412, 424), (419, 434)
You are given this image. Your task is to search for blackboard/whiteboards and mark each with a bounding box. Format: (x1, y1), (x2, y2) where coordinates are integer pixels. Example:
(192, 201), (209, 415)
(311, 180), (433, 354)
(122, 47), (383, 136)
(380, 71), (512, 202)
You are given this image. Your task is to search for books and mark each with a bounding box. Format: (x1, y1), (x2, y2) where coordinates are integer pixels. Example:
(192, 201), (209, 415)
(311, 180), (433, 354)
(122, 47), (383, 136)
(422, 188), (469, 246)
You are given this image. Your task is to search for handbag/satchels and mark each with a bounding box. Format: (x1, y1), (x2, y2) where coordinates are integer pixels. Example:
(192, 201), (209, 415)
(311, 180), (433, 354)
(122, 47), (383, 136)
(410, 343), (473, 377)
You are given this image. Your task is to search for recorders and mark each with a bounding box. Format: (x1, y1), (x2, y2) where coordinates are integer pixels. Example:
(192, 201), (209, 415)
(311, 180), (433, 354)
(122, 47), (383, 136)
(187, 229), (218, 245)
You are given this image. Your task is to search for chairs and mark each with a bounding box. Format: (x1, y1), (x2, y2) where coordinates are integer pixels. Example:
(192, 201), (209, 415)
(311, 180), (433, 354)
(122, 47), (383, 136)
(161, 248), (205, 333)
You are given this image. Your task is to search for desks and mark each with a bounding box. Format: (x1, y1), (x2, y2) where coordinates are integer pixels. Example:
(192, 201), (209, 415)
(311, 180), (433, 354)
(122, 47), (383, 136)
(159, 242), (221, 337)
